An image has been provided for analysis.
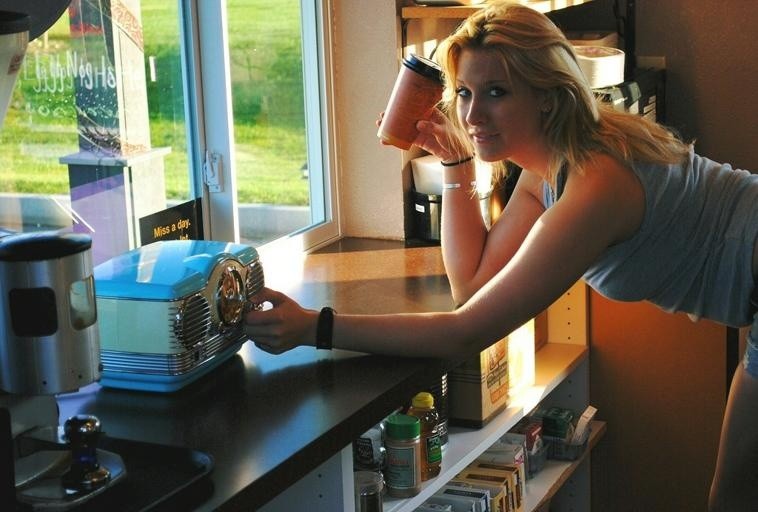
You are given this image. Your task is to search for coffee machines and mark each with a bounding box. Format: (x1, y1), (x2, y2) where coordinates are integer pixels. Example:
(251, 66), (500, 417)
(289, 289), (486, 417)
(0, 226), (217, 512)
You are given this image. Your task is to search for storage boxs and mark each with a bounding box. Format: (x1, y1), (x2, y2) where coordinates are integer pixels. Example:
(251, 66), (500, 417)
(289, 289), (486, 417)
(410, 154), (495, 197)
(446, 336), (511, 428)
(568, 40), (626, 89)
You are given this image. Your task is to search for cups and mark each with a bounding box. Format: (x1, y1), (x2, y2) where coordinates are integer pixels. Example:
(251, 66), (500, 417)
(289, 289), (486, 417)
(376, 51), (447, 151)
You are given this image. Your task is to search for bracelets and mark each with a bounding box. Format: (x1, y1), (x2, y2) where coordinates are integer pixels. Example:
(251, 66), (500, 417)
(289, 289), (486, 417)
(443, 181), (478, 188)
(317, 309), (334, 350)
(441, 155), (473, 168)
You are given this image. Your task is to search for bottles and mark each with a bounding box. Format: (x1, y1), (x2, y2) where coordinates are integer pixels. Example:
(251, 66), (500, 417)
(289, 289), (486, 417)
(354, 470), (384, 512)
(407, 391), (443, 481)
(422, 375), (451, 452)
(354, 423), (385, 474)
(385, 416), (422, 498)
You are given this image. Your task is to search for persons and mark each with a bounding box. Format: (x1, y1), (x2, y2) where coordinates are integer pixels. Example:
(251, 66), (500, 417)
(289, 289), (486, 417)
(243, 1), (758, 510)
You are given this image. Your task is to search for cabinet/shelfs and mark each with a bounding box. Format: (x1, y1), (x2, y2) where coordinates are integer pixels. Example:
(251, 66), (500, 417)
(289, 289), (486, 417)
(3, 232), (601, 512)
(394, 3), (665, 238)
